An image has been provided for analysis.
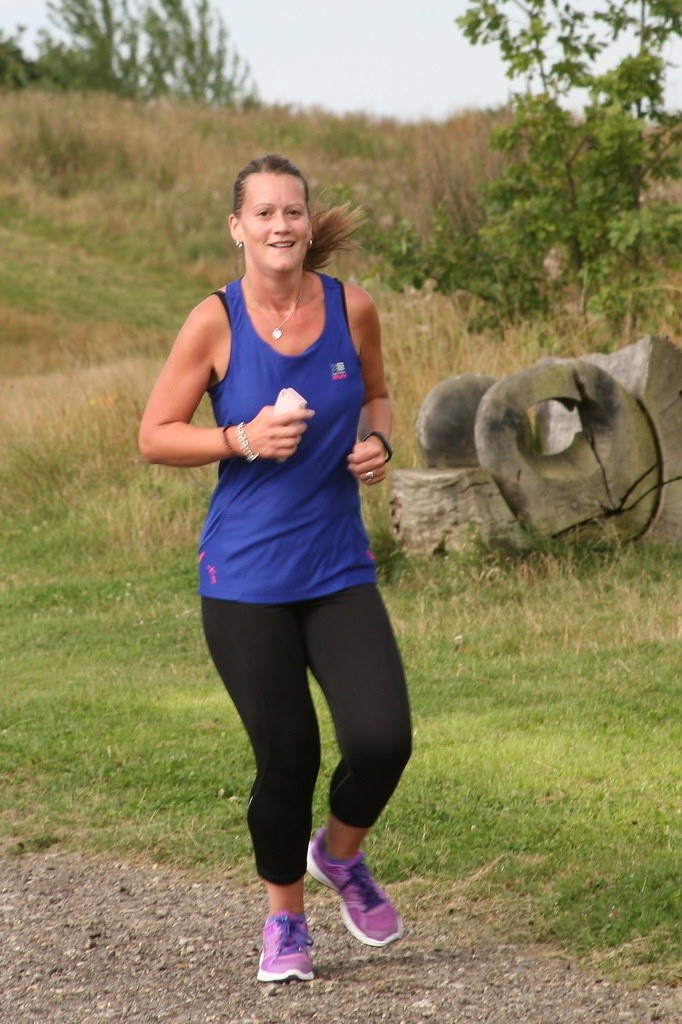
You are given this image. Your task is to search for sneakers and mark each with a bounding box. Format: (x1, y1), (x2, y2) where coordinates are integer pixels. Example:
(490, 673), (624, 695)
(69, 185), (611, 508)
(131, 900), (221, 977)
(259, 912), (314, 981)
(306, 830), (406, 949)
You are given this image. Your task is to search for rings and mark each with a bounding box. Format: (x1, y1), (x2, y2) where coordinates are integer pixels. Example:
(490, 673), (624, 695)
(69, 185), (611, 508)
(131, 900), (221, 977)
(367, 471), (374, 480)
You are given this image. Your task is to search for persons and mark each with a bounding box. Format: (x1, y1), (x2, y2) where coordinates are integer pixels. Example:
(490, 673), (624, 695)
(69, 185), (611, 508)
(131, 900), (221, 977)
(136, 156), (415, 983)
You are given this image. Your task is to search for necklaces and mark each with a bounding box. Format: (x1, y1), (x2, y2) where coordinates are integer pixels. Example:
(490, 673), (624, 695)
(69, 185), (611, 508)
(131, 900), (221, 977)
(242, 274), (305, 339)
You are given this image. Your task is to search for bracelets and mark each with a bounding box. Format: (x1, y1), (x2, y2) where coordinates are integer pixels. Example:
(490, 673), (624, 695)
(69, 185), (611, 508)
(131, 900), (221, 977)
(237, 422), (259, 464)
(360, 430), (394, 463)
(223, 424), (238, 458)
